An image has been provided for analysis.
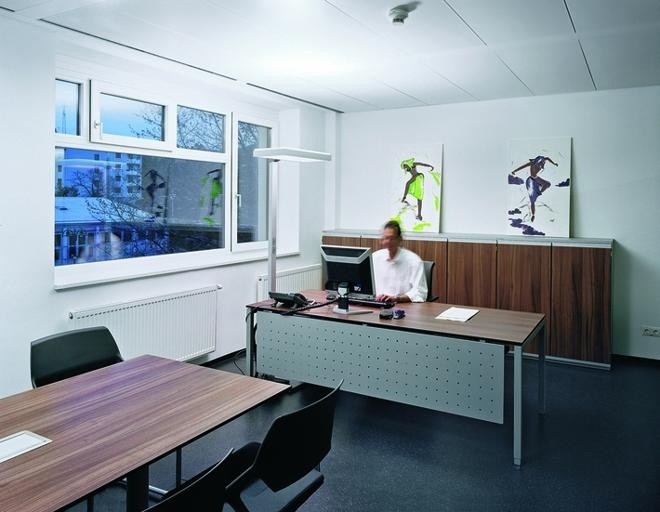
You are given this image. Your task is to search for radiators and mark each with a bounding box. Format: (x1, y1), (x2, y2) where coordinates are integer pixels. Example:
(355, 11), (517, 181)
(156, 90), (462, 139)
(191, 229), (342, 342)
(69, 285), (222, 362)
(256, 264), (321, 302)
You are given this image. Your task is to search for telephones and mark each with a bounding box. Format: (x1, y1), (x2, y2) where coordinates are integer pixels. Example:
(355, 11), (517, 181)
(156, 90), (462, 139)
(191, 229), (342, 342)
(268, 291), (309, 310)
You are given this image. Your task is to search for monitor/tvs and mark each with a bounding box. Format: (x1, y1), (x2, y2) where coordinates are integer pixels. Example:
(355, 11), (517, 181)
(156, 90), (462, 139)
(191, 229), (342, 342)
(320, 245), (377, 316)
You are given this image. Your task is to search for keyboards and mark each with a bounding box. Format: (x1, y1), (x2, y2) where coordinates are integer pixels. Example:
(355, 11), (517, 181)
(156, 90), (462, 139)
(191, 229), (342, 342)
(342, 292), (395, 309)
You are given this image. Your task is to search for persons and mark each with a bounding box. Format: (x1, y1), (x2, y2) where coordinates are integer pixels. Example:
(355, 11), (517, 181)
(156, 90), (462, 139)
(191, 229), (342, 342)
(143, 169), (166, 208)
(207, 168), (223, 216)
(512, 156), (559, 221)
(371, 221), (429, 303)
(401, 159), (433, 220)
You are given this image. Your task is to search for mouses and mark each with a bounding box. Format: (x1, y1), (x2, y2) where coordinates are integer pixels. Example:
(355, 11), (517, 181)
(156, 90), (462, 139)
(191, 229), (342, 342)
(326, 294), (335, 300)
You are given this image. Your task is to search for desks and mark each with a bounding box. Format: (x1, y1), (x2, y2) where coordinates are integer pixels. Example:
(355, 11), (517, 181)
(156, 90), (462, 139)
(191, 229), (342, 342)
(245, 290), (548, 466)
(0, 354), (292, 512)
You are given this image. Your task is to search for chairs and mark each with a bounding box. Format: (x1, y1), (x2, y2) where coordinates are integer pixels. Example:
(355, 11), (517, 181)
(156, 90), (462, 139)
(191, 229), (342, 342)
(29, 326), (181, 512)
(161, 379), (343, 512)
(143, 448), (234, 512)
(422, 261), (439, 302)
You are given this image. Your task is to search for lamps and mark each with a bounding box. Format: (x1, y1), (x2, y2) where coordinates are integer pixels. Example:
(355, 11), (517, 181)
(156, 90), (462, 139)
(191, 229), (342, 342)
(387, 8), (408, 26)
(249, 146), (332, 298)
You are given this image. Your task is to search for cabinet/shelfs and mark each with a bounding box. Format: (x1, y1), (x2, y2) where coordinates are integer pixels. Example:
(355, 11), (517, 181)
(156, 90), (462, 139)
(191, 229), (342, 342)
(321, 229), (613, 371)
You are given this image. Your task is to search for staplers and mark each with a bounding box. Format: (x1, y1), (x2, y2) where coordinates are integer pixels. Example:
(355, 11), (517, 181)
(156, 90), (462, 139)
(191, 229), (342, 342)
(394, 308), (406, 320)
